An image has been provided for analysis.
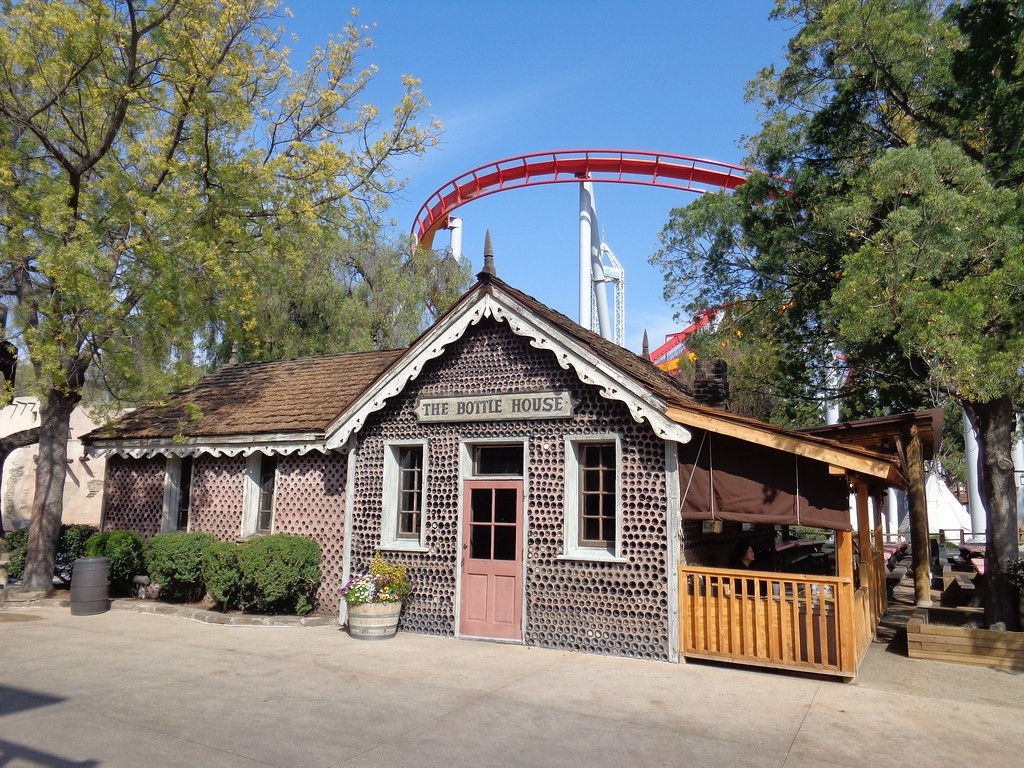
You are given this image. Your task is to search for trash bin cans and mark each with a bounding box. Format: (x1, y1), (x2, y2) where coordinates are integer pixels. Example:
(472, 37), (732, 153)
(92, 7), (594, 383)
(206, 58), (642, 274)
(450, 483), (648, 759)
(69, 557), (113, 615)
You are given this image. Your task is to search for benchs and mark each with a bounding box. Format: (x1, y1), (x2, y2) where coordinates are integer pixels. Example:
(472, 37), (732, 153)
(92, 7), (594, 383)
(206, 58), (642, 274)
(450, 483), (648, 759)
(956, 575), (975, 590)
(886, 555), (913, 583)
(788, 547), (834, 577)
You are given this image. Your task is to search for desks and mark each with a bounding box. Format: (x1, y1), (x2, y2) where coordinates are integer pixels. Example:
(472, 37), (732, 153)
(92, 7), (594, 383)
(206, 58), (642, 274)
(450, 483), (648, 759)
(963, 545), (985, 577)
(775, 540), (826, 571)
(883, 541), (909, 577)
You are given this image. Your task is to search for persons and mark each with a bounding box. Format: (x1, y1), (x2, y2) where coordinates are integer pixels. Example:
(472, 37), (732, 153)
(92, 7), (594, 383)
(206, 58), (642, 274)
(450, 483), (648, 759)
(959, 547), (976, 572)
(888, 547), (909, 570)
(722, 541), (781, 595)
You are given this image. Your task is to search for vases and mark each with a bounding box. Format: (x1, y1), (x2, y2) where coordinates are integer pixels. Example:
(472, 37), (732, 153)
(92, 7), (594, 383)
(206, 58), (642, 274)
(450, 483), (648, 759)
(346, 601), (401, 639)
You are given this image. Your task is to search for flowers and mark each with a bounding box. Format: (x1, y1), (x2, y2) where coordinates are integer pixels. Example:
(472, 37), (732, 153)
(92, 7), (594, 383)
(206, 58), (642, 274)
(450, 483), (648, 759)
(341, 547), (417, 606)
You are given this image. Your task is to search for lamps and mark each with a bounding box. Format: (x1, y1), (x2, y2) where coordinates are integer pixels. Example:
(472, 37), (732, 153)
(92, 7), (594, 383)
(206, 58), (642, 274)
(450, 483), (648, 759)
(880, 431), (892, 448)
